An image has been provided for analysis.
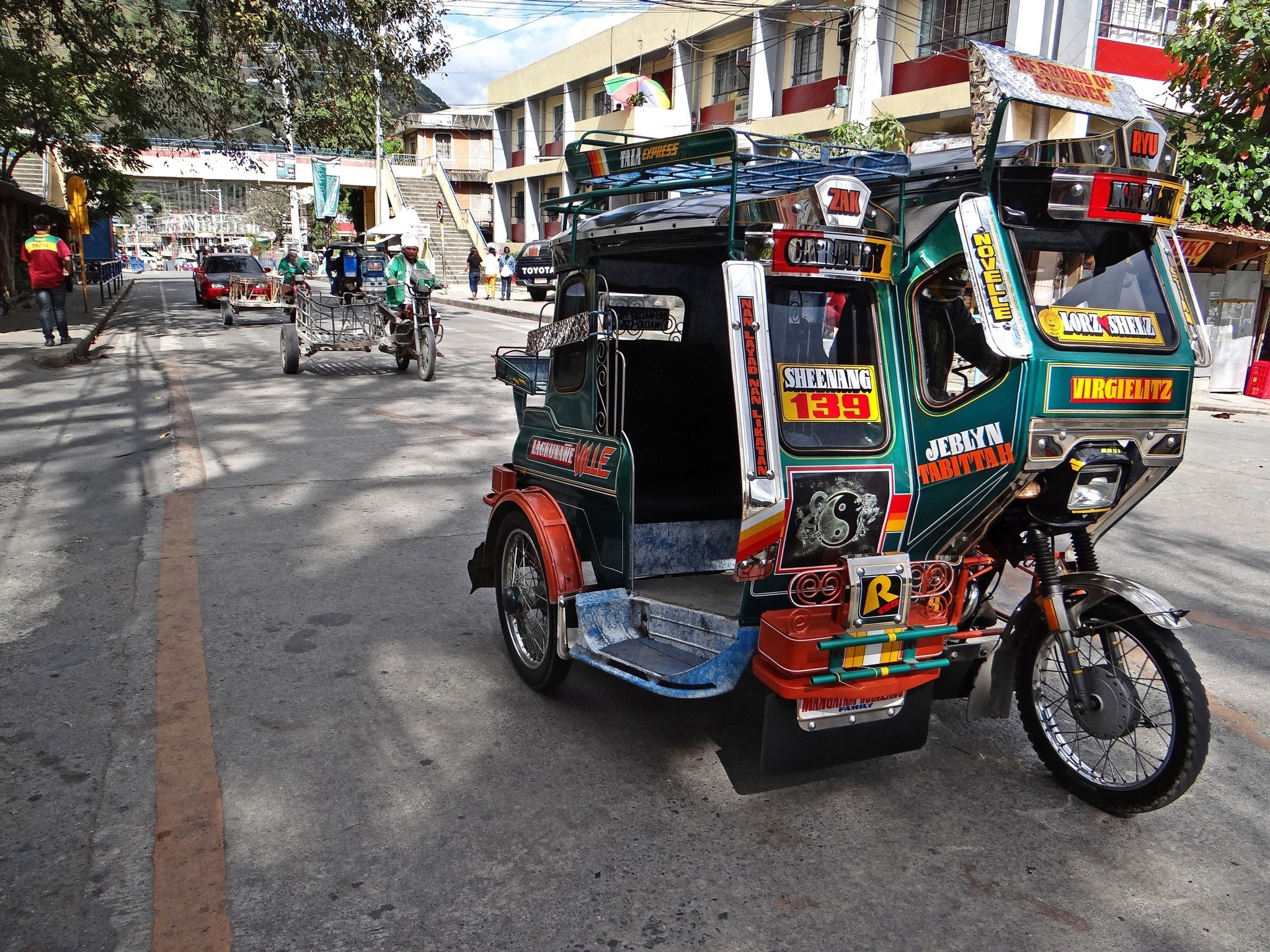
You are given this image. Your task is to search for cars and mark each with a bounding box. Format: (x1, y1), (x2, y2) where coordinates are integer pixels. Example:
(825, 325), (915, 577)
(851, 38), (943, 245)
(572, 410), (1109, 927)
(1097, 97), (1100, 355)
(191, 253), (274, 309)
(514, 238), (557, 302)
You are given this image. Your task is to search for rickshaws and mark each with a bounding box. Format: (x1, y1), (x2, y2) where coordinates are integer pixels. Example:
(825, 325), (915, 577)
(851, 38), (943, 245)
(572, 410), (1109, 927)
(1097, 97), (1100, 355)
(278, 268), (444, 382)
(465, 39), (1215, 818)
(322, 241), (391, 307)
(219, 265), (315, 327)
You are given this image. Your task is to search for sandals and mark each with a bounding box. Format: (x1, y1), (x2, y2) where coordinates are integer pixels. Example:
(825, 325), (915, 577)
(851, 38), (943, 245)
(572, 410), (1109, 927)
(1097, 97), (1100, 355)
(436, 351), (445, 358)
(390, 346), (398, 352)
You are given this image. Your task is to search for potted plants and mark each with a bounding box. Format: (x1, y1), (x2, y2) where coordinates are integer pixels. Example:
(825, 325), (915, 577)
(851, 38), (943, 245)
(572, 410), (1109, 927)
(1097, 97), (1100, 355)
(508, 252), (518, 283)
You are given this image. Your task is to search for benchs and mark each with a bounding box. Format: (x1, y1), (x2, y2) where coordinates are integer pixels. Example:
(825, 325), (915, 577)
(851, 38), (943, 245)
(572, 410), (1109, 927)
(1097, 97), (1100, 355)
(607, 339), (765, 573)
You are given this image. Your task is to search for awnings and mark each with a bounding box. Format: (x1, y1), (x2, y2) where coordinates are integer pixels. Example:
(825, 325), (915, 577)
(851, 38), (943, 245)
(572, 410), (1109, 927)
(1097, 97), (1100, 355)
(224, 237), (269, 247)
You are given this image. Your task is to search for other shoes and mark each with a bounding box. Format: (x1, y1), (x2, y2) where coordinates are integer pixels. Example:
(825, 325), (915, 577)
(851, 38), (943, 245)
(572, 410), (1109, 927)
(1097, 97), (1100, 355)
(973, 592), (996, 626)
(484, 294), (490, 300)
(507, 298), (511, 300)
(491, 297), (497, 300)
(44, 339), (55, 346)
(472, 292), (477, 301)
(60, 336), (73, 343)
(500, 297), (504, 300)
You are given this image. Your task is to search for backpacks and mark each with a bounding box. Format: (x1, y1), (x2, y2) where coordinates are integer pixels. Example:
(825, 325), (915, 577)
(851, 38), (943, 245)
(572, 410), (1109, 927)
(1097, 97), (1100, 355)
(500, 256), (512, 277)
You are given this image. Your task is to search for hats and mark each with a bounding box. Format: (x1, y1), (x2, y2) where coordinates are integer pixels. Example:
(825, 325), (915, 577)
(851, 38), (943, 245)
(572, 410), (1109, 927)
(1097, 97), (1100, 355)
(400, 233), (420, 250)
(288, 244), (300, 252)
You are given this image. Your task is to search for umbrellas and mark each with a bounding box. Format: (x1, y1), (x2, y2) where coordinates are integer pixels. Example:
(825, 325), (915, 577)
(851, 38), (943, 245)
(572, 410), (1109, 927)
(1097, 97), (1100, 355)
(604, 72), (671, 110)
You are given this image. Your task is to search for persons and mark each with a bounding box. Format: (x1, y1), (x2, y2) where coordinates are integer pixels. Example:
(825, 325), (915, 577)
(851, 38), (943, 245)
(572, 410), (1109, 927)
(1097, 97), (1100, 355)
(114, 248), (128, 269)
(308, 249), (327, 275)
(384, 233), (449, 359)
(277, 243), (313, 314)
(464, 246), (516, 301)
(328, 249), (349, 296)
(612, 103), (622, 112)
(827, 262), (1028, 403)
(20, 213), (74, 347)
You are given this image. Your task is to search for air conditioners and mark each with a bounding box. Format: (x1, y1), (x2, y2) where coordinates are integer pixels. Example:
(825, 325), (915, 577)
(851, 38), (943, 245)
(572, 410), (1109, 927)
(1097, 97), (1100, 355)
(736, 47), (750, 67)
